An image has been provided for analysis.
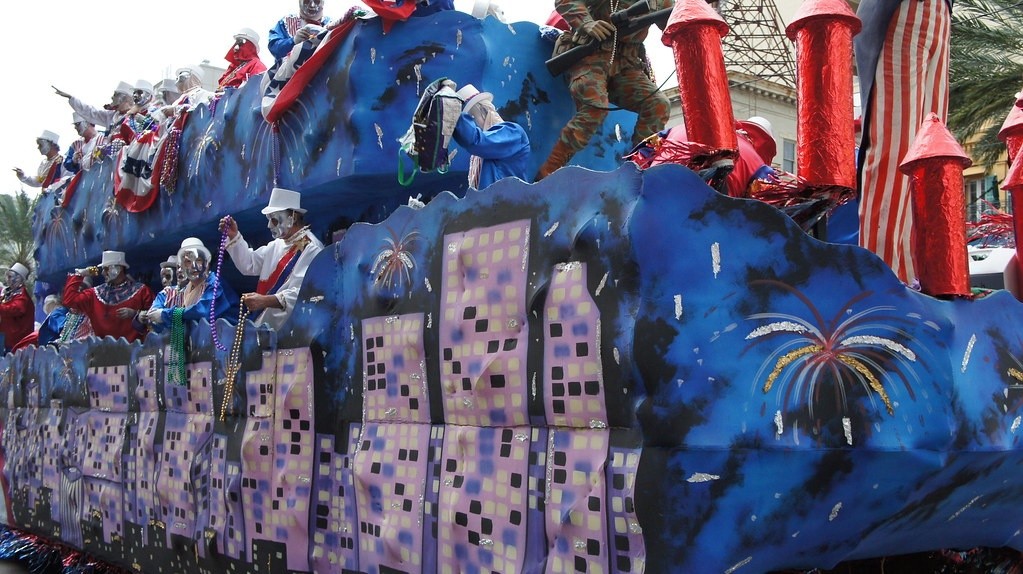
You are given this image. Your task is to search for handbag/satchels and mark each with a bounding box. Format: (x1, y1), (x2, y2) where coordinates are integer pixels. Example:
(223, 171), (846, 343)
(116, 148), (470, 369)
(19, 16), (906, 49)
(396, 77), (464, 187)
(551, 30), (573, 59)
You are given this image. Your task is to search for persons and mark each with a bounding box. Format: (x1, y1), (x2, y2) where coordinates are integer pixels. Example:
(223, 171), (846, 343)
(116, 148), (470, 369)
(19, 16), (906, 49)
(219, 188), (324, 342)
(0, 262), (59, 356)
(62, 250), (152, 344)
(48, 268), (93, 350)
(534, 0), (676, 187)
(441, 79), (530, 191)
(12, 0), (458, 187)
(739, 114), (777, 164)
(138, 236), (246, 342)
(854, 0), (955, 288)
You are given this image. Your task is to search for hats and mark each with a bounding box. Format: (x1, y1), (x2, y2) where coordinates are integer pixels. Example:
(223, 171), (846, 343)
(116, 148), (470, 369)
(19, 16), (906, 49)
(9, 262), (29, 281)
(455, 83), (493, 115)
(133, 80), (154, 96)
(177, 237), (212, 263)
(261, 187), (308, 215)
(114, 82), (135, 97)
(37, 130), (60, 151)
(158, 79), (180, 93)
(71, 112), (88, 123)
(159, 255), (180, 267)
(97, 251), (129, 269)
(174, 65), (205, 81)
(739, 116), (776, 147)
(233, 29), (260, 53)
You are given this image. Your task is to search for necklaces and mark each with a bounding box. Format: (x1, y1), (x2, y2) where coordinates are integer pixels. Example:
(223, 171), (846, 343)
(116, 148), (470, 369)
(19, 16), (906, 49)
(137, 111), (162, 141)
(41, 156), (55, 174)
(167, 307), (188, 389)
(177, 284), (180, 303)
(60, 312), (85, 341)
(211, 214), (230, 351)
(610, 0), (618, 64)
(220, 62), (247, 85)
(152, 116), (178, 149)
(220, 295), (250, 423)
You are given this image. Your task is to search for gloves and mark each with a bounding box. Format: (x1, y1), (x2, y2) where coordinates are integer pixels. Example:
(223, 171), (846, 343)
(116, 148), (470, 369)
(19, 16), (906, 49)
(581, 18), (616, 41)
